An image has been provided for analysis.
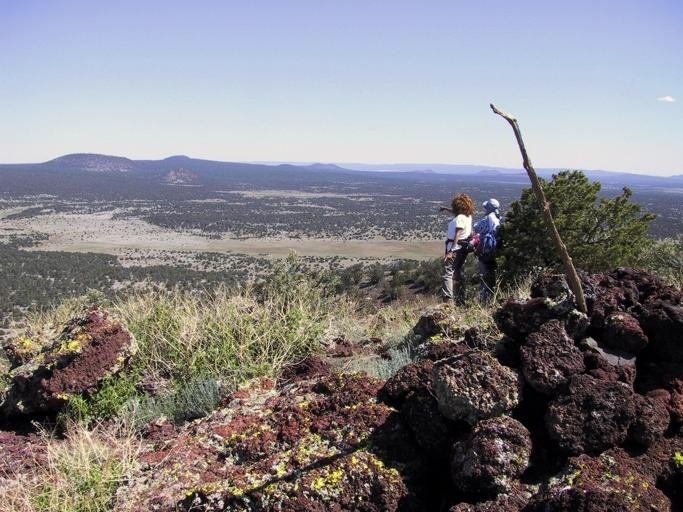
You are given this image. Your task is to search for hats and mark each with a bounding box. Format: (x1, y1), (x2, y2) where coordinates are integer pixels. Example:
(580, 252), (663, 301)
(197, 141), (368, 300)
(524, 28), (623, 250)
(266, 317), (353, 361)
(482, 199), (500, 215)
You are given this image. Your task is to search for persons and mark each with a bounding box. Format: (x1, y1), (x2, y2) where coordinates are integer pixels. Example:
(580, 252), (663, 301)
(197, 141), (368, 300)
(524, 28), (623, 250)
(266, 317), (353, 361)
(438, 193), (473, 303)
(473, 199), (502, 301)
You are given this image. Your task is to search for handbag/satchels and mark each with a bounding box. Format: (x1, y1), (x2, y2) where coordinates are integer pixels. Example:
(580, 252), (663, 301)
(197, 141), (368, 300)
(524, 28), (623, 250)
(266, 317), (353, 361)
(461, 235), (480, 253)
(476, 233), (496, 257)
